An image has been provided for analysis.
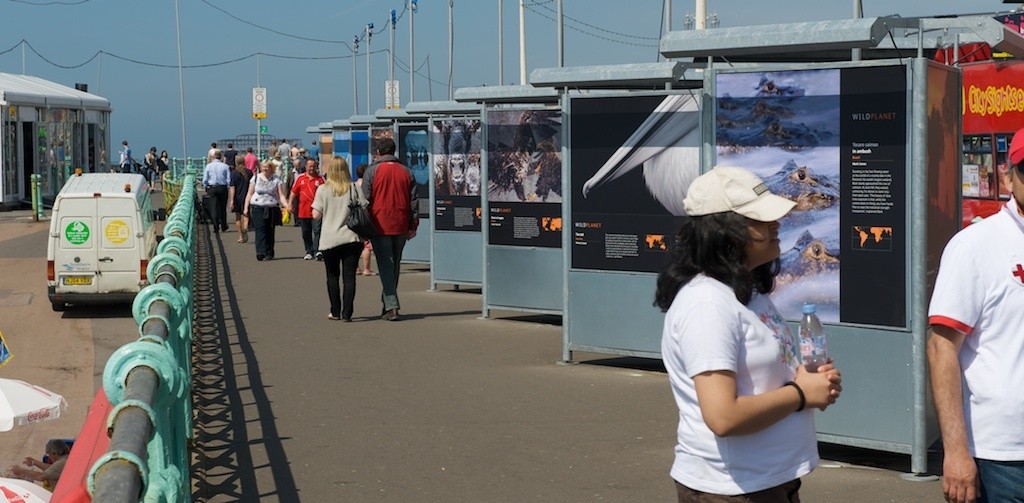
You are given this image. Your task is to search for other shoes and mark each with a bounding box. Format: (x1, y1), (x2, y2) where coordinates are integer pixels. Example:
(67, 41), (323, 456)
(303, 253), (312, 260)
(355, 265), (362, 275)
(363, 270), (378, 276)
(222, 224), (228, 231)
(316, 252), (323, 261)
(343, 317), (352, 322)
(327, 310), (340, 320)
(238, 231), (248, 243)
(256, 252), (273, 260)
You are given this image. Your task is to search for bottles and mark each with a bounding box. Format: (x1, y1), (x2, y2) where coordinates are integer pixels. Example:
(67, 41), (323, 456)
(799, 303), (827, 373)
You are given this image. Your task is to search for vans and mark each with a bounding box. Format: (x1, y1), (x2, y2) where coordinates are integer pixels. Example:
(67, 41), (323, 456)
(46, 168), (167, 312)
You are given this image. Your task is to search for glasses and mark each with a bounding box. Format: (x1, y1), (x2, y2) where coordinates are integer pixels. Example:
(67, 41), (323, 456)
(1010, 159), (1024, 173)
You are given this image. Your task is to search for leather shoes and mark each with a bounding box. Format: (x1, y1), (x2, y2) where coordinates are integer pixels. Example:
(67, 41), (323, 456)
(380, 306), (398, 320)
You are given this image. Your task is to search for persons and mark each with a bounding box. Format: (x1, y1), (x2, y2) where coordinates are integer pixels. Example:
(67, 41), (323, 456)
(202, 138), (319, 243)
(10, 439), (70, 493)
(243, 156), (325, 261)
(50, 140), (71, 189)
(311, 137), (419, 324)
(144, 147), (169, 193)
(98, 141), (107, 168)
(118, 141), (137, 173)
(653, 165), (843, 503)
(927, 125), (1024, 503)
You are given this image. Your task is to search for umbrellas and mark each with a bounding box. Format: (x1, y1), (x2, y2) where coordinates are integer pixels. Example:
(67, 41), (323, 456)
(0, 378), (69, 432)
(0, 477), (53, 503)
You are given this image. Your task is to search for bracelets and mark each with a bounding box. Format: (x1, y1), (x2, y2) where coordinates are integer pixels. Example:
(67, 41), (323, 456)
(784, 381), (805, 411)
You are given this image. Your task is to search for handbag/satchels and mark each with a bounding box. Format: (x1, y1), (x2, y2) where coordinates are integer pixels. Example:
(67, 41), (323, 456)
(347, 183), (376, 240)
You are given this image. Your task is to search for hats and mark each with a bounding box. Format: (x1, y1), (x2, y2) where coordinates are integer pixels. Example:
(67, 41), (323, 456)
(1009, 127), (1024, 166)
(683, 165), (800, 222)
